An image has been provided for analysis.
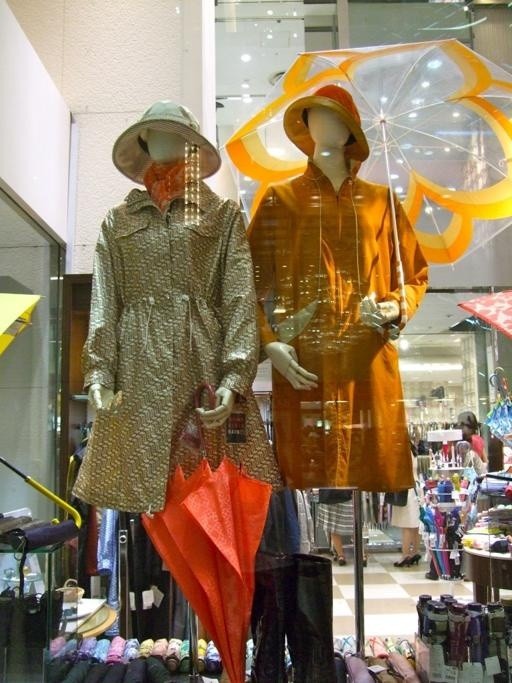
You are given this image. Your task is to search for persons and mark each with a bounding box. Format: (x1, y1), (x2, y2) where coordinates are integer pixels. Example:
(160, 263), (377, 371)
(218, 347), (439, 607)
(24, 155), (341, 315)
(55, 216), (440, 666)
(246, 86), (428, 493)
(390, 445), (422, 568)
(318, 488), (369, 567)
(72, 101), (281, 512)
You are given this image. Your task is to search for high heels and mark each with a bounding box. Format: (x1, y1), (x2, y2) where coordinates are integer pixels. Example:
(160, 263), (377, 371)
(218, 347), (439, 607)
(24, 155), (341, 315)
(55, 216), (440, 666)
(392, 557), (410, 568)
(333, 553), (369, 567)
(408, 554), (421, 566)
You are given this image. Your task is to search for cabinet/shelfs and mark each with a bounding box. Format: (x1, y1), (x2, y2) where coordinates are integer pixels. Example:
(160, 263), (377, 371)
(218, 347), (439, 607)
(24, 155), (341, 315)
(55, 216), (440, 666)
(423, 465), (469, 553)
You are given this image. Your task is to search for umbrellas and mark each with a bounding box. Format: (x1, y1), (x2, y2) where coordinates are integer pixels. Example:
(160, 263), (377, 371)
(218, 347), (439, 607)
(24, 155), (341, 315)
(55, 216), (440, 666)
(219, 38), (512, 337)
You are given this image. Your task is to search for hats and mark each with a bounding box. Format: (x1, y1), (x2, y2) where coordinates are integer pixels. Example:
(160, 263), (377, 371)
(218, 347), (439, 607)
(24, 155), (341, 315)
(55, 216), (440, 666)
(282, 84), (369, 162)
(111, 99), (222, 186)
(454, 410), (481, 428)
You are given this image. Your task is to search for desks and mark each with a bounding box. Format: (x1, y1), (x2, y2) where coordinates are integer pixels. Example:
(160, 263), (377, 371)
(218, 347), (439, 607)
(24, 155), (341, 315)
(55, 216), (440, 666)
(460, 528), (512, 605)
